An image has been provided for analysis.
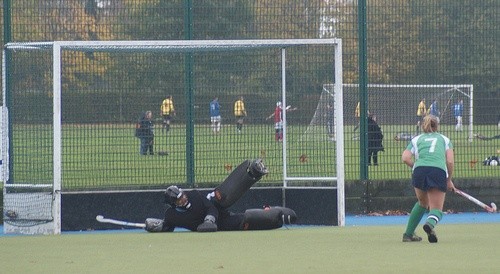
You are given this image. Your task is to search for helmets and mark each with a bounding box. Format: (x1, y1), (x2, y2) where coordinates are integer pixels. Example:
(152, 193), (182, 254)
(164, 186), (184, 208)
(277, 101), (282, 107)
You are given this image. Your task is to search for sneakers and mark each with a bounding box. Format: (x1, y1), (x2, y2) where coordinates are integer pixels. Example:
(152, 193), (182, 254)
(423, 222), (438, 243)
(403, 232), (422, 241)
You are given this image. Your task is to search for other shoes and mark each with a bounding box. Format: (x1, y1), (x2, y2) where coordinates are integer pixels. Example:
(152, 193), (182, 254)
(279, 207), (297, 224)
(374, 163), (378, 166)
(368, 163), (371, 165)
(248, 159), (268, 180)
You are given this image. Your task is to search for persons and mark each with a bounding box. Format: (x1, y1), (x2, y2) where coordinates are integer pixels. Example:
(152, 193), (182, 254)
(367, 113), (385, 166)
(159, 94), (175, 134)
(414, 98), (464, 133)
(472, 121), (500, 164)
(265, 101), (297, 143)
(144, 158), (297, 232)
(208, 94), (222, 137)
(324, 98), (370, 139)
(234, 95), (248, 132)
(135, 110), (154, 155)
(402, 114), (455, 243)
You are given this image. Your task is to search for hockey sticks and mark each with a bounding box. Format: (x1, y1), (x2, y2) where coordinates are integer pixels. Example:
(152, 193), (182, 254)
(96, 215), (146, 228)
(455, 188), (497, 213)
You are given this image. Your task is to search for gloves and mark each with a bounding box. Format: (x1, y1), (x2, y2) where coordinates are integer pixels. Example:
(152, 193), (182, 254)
(197, 221), (217, 232)
(145, 218), (163, 231)
